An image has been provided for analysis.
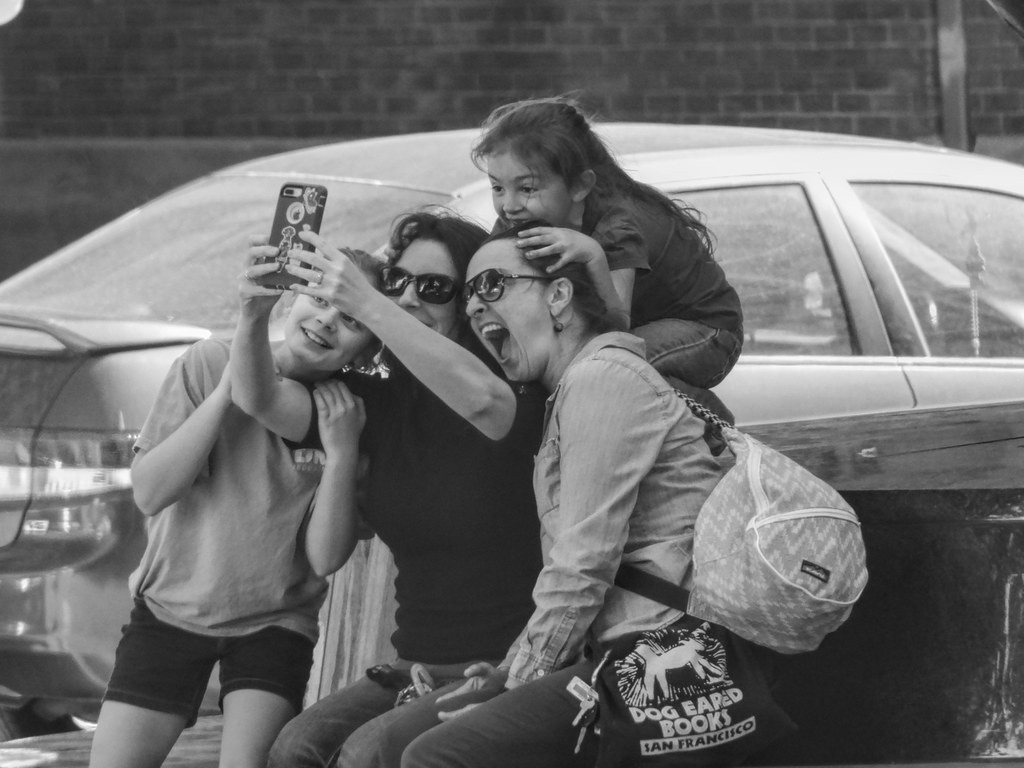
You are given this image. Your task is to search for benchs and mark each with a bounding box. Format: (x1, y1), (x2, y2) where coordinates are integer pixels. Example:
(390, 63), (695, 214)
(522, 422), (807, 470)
(722, 486), (1024, 768)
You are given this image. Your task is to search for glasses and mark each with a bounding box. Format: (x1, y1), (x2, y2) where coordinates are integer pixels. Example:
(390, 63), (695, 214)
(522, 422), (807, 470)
(455, 268), (553, 314)
(377, 266), (463, 306)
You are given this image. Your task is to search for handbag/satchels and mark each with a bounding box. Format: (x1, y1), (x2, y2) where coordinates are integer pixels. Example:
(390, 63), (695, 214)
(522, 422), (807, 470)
(602, 345), (869, 655)
(582, 617), (800, 768)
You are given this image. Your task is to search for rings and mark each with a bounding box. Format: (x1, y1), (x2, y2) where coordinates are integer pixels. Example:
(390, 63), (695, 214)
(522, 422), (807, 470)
(244, 269), (252, 279)
(315, 272), (324, 285)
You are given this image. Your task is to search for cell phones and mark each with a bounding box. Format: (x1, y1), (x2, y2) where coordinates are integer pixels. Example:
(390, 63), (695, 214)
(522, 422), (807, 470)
(262, 183), (328, 290)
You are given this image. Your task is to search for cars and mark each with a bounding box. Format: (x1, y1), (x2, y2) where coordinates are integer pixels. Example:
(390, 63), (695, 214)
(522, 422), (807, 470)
(0, 124), (1024, 733)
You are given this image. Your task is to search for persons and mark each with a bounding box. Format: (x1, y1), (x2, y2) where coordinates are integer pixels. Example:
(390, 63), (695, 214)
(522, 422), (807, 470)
(88, 248), (386, 768)
(224, 205), (542, 768)
(399, 219), (774, 768)
(474, 97), (747, 426)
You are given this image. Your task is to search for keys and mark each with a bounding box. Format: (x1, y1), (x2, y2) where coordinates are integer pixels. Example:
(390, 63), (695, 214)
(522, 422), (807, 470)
(572, 697), (595, 727)
(574, 706), (598, 754)
